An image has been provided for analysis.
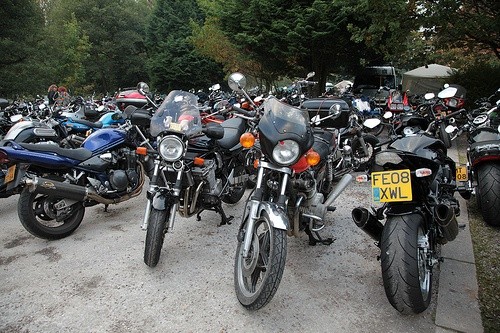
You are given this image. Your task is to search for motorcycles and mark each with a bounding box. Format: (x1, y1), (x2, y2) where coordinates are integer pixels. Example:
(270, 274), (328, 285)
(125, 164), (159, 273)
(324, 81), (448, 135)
(131, 80), (251, 267)
(451, 100), (500, 226)
(351, 88), (469, 316)
(1, 102), (157, 241)
(227, 73), (352, 311)
(1, 89), (493, 177)
